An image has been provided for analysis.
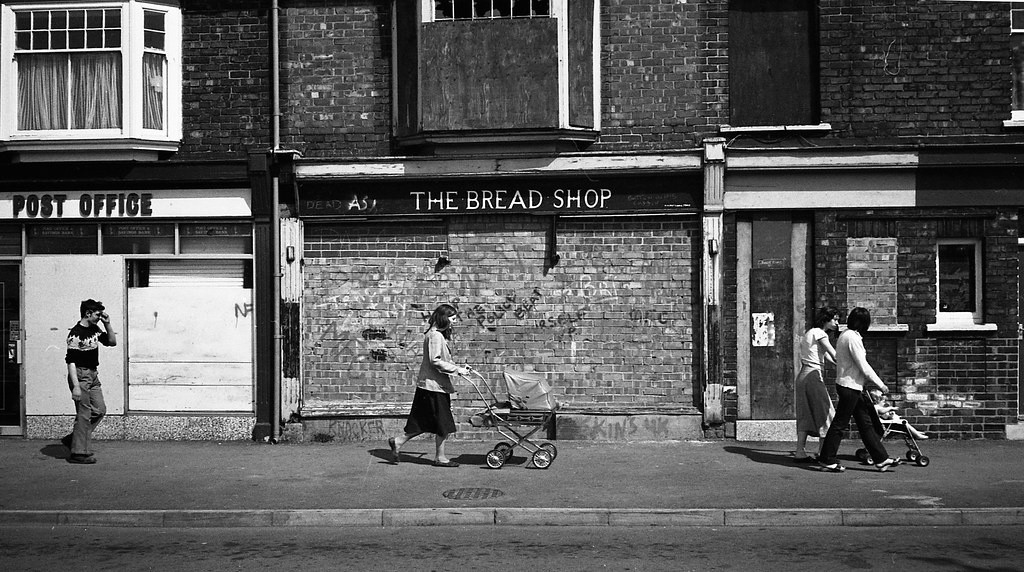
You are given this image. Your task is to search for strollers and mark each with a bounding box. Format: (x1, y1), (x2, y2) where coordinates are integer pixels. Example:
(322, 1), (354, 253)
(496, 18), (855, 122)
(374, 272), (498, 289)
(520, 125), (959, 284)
(854, 388), (930, 467)
(458, 366), (561, 470)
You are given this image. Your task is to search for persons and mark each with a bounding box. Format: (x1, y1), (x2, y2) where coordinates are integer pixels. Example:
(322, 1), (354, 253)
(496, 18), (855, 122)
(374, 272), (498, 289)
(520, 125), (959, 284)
(389, 304), (472, 467)
(869, 388), (929, 440)
(818, 307), (903, 472)
(61, 300), (117, 464)
(794, 306), (841, 463)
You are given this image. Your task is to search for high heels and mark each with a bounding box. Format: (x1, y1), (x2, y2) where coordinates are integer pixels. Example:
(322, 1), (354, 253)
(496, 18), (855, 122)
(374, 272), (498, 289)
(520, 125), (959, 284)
(822, 463), (845, 472)
(877, 458), (901, 472)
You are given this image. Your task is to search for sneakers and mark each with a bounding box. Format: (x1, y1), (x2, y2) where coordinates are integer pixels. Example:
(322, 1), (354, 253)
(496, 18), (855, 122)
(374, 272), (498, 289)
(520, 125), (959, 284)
(432, 460), (460, 467)
(388, 437), (400, 465)
(69, 453), (97, 464)
(61, 435), (72, 451)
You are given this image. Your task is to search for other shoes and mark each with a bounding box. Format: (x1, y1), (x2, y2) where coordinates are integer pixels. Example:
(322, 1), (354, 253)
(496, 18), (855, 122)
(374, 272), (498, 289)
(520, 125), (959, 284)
(793, 455), (816, 463)
